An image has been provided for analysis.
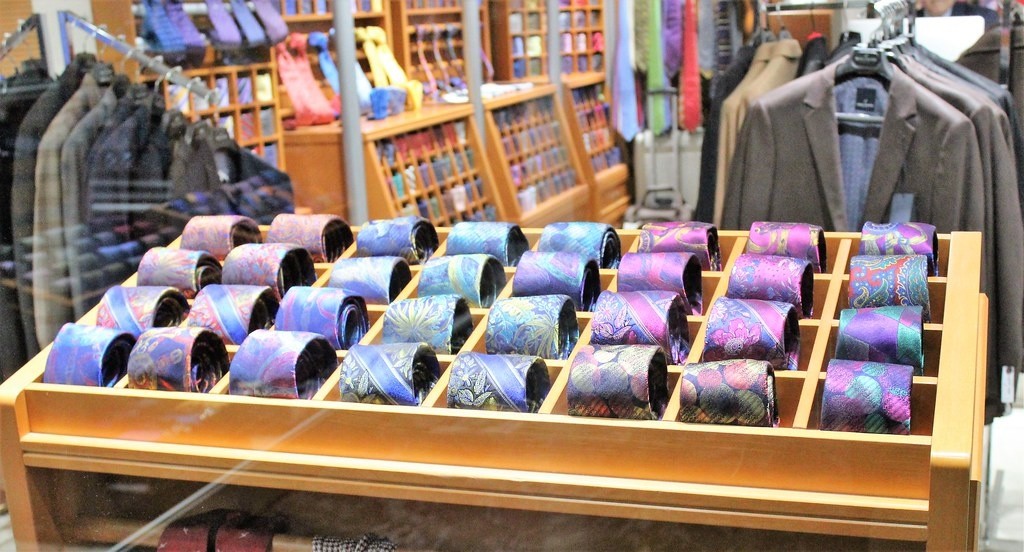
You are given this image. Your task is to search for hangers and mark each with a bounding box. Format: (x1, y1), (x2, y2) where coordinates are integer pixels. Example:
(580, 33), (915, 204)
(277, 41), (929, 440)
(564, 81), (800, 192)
(744, 1), (1010, 137)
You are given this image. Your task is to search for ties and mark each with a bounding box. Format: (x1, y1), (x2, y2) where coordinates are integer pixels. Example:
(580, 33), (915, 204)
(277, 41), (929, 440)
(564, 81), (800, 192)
(41, 0), (939, 435)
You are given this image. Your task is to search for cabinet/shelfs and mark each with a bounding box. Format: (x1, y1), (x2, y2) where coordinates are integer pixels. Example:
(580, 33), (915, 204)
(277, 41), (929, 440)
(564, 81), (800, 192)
(132, 1), (632, 226)
(0, 225), (987, 552)
